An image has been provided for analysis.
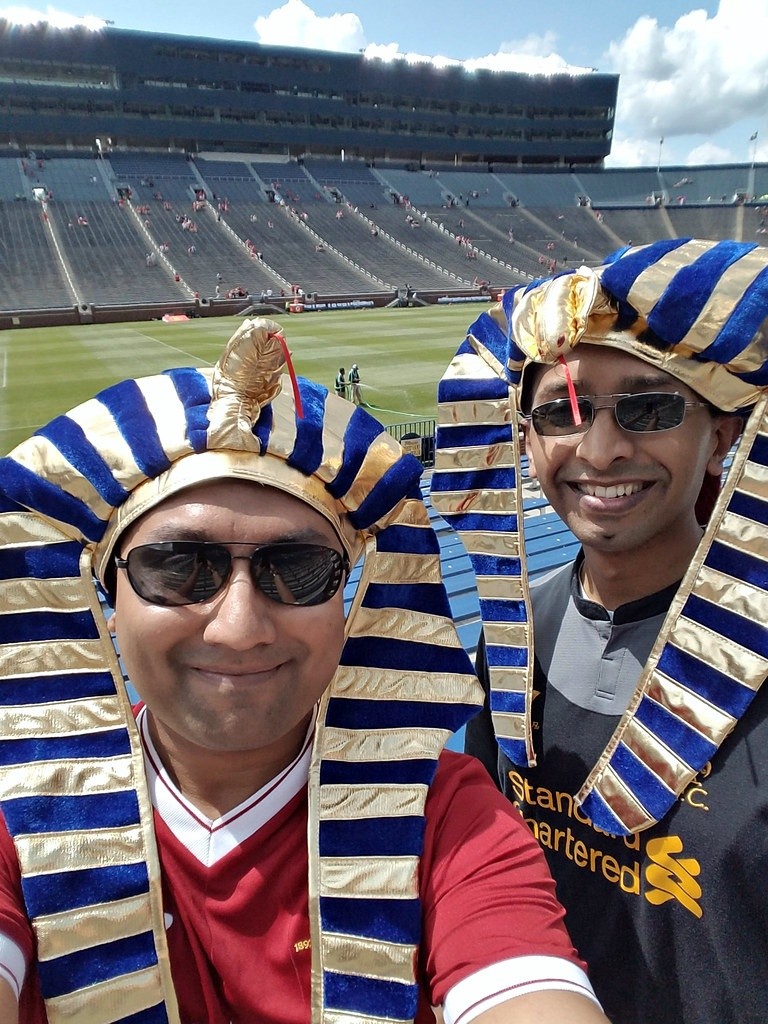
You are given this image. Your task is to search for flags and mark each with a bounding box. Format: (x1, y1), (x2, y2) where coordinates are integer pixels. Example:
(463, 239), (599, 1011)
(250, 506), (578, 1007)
(750, 131), (757, 140)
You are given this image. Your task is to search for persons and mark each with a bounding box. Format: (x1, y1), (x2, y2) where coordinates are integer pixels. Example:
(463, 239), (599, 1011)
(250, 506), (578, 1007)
(175, 273), (180, 285)
(474, 188), (604, 287)
(0, 317), (610, 1024)
(68, 216), (90, 230)
(261, 284), (302, 297)
(118, 178), (198, 234)
(146, 242), (197, 267)
(168, 551), (223, 604)
(354, 170), (479, 264)
(215, 273), (249, 298)
(192, 189), (207, 212)
(256, 557), (295, 604)
(646, 177), (768, 236)
(334, 367), (350, 399)
(348, 364), (365, 405)
(432, 238), (768, 1023)
(212, 183), (344, 260)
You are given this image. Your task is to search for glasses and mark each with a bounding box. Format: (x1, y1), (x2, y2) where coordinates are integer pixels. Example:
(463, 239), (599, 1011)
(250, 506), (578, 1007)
(114, 541), (349, 607)
(526, 391), (711, 436)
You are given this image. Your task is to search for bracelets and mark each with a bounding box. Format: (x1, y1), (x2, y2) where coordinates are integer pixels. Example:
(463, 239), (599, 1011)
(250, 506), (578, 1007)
(210, 567), (218, 574)
(272, 570), (279, 577)
